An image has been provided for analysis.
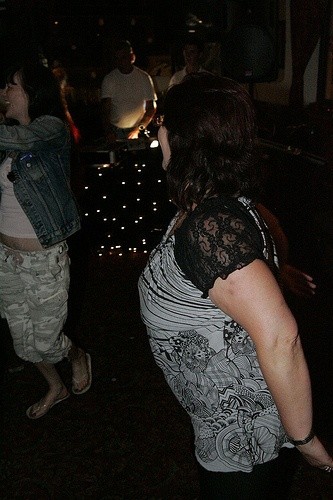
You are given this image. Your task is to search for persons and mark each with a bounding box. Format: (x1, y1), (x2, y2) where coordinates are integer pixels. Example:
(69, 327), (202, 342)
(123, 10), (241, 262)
(138, 72), (333, 500)
(0, 59), (92, 419)
(52, 32), (216, 262)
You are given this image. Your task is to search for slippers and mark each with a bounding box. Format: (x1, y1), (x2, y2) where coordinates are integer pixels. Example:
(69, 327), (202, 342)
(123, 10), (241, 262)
(72, 352), (92, 395)
(26, 392), (71, 420)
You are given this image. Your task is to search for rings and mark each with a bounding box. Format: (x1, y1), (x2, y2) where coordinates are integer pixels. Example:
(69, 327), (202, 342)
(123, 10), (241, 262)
(322, 466), (331, 473)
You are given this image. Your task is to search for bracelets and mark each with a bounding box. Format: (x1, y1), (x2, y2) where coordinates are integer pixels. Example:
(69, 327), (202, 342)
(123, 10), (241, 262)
(289, 429), (315, 446)
